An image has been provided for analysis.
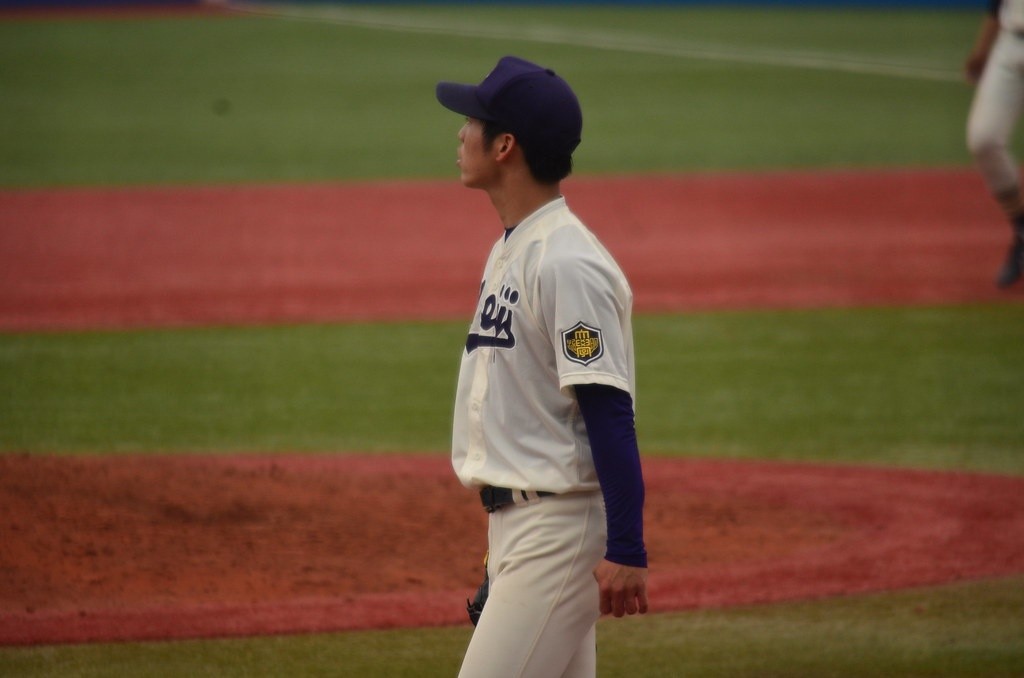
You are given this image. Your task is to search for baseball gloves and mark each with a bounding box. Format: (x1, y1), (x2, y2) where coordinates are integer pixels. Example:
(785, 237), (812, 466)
(464, 546), (489, 629)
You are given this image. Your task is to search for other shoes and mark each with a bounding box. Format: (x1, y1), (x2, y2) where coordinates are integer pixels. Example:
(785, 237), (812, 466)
(998, 230), (1024, 286)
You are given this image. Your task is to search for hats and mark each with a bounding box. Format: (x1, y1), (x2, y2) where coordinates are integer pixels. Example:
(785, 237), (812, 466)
(436, 54), (582, 160)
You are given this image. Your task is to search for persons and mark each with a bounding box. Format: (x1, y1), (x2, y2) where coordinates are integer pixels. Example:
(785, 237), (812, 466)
(963, 0), (1024, 287)
(437, 56), (648, 678)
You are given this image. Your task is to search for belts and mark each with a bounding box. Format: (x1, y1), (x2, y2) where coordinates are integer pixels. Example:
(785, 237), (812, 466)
(479, 480), (554, 513)
